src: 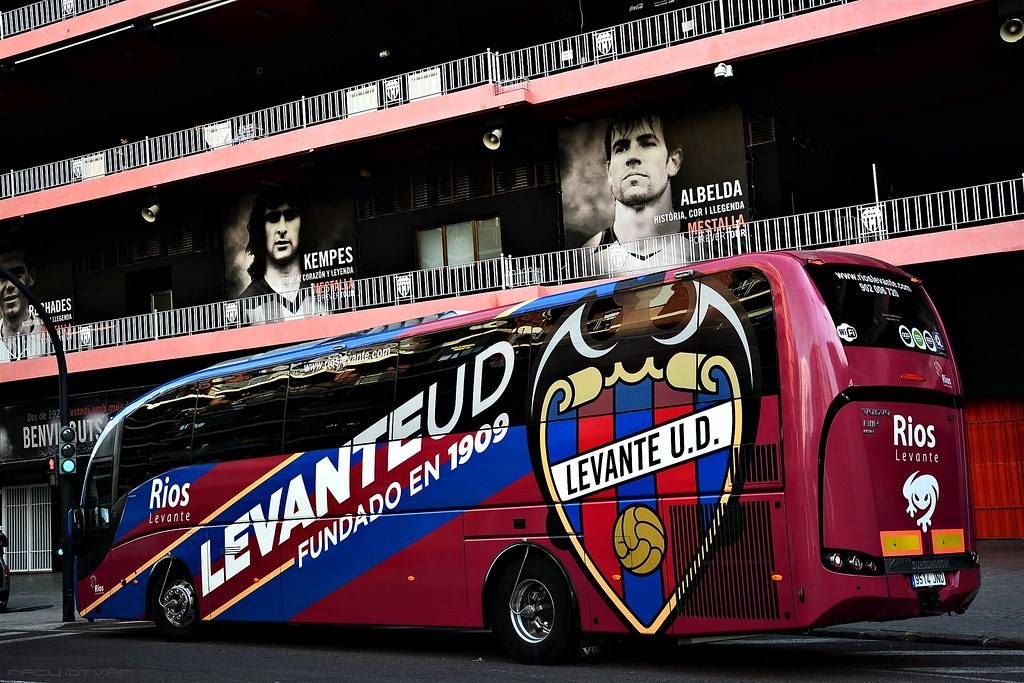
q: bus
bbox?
[67,250,982,663]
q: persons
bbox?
[575,104,716,279]
[238,184,332,326]
[0,250,46,363]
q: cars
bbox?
[0,530,10,609]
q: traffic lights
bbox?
[46,457,59,486]
[58,427,78,474]
[56,539,73,565]
